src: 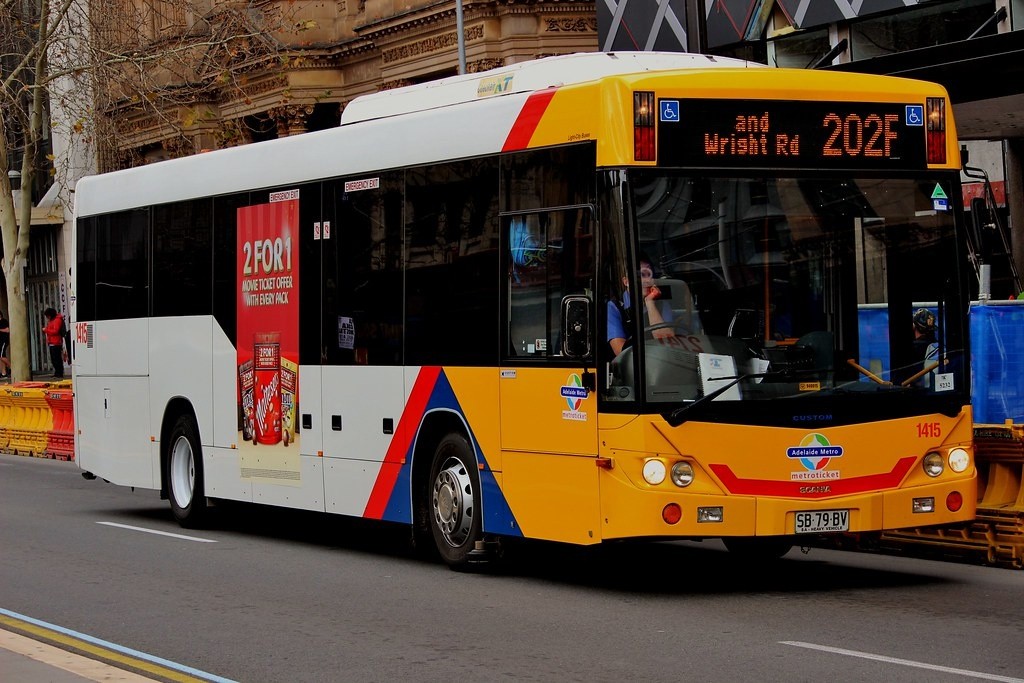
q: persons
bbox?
[913,309,948,389]
[605,260,674,356]
[0,311,12,378]
[41,308,67,381]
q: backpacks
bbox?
[58,318,66,337]
[921,341,947,389]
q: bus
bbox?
[70,51,1012,573]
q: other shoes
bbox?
[50,375,65,380]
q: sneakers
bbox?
[1,374,7,381]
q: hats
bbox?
[913,308,940,330]
